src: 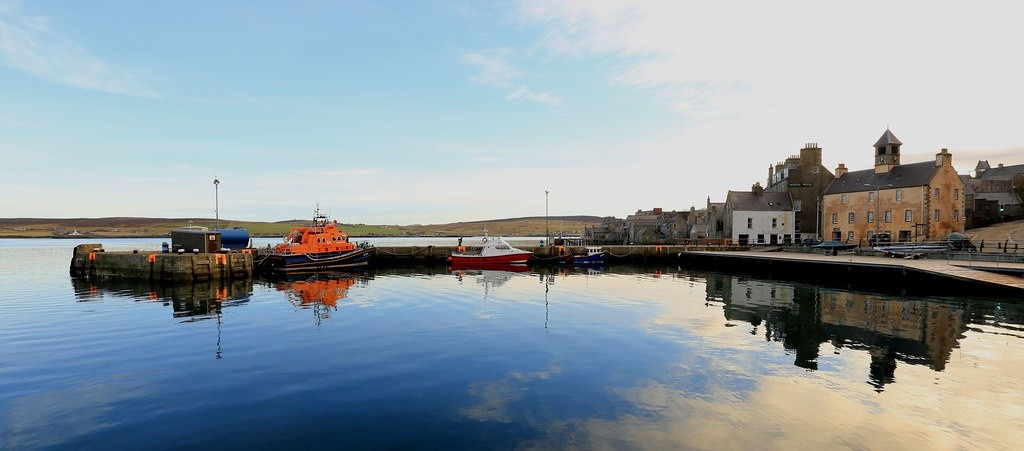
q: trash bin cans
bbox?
[162,242,169,253]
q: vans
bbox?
[870,233,891,247]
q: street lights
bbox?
[545,190,551,247]
[213,178,220,231]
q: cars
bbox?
[804,238,821,245]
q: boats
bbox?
[447,263,532,276]
[572,246,609,268]
[526,246,575,269]
[250,203,372,277]
[445,227,534,266]
